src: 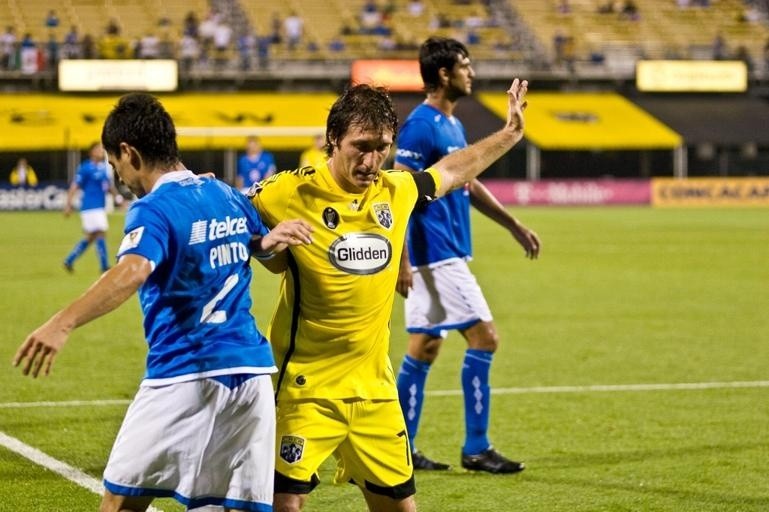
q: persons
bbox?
[64,142,129,275]
[1,1,769,216]
[14,95,289,512]
[392,37,542,473]
[245,78,528,510]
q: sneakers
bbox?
[412,448,449,469]
[461,444,525,473]
